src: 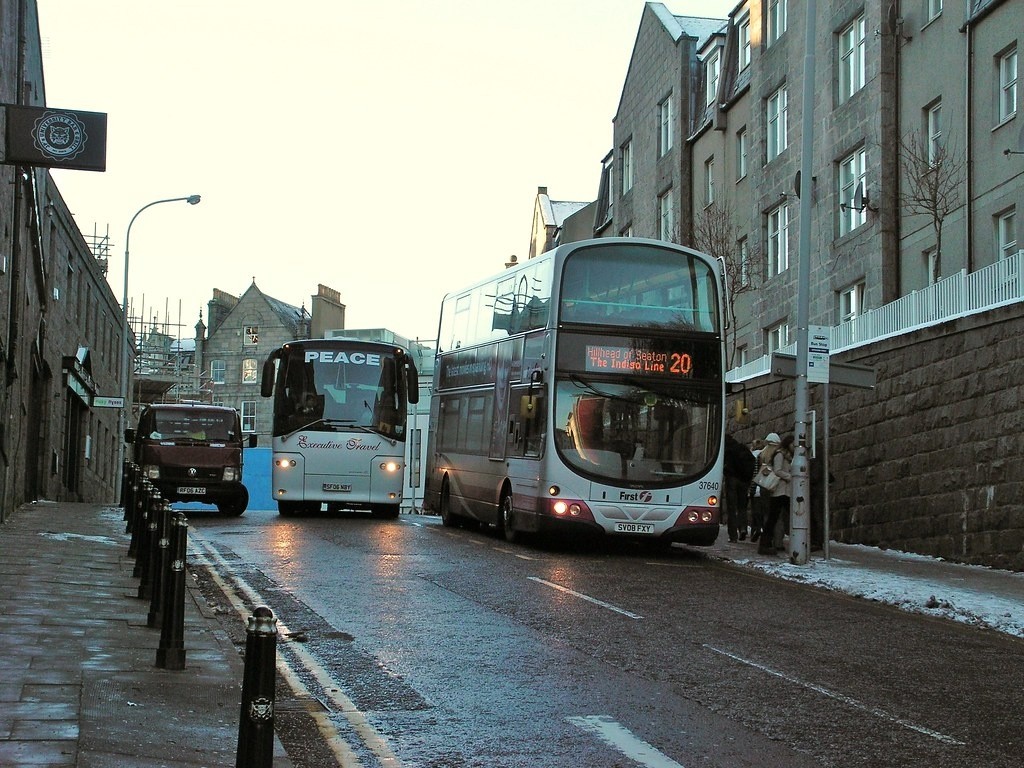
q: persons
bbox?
[724,432,756,542]
[750,433,795,555]
[298,395,318,416]
[810,441,834,553]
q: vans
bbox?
[125,403,257,517]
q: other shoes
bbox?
[809,541,823,552]
[775,547,786,551]
[728,537,737,543]
[739,529,747,541]
[758,545,777,555]
[751,529,761,542]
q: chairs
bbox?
[562,297,661,324]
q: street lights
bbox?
[113,195,201,504]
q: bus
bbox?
[260,337,418,520]
[423,236,749,560]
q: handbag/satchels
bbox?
[754,450,784,492]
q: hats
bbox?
[765,432,781,443]
